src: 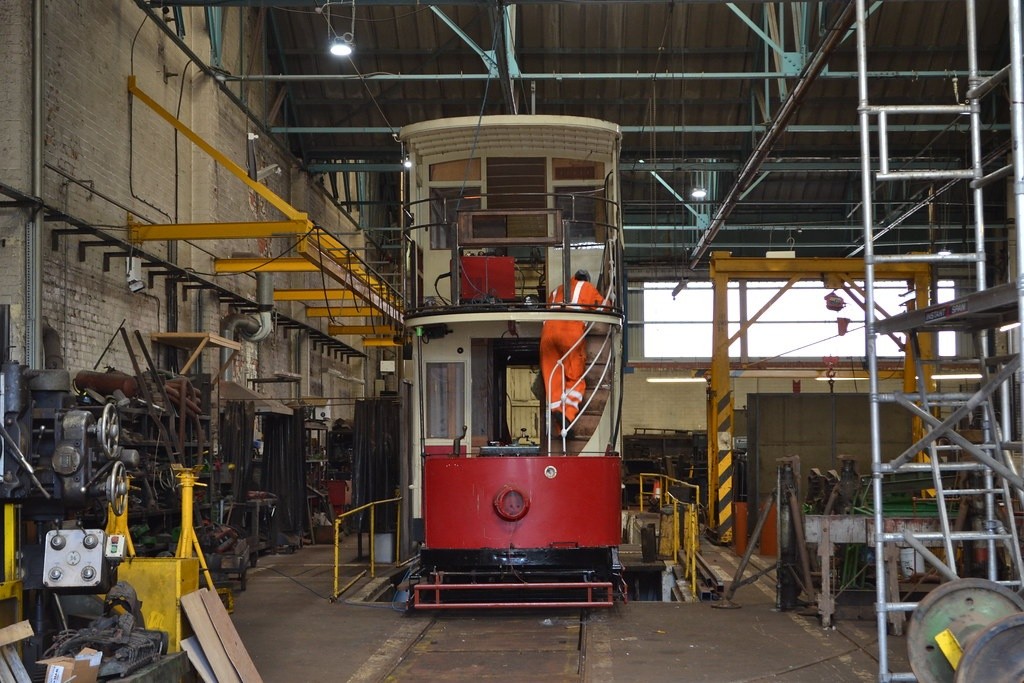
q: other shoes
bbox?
[554,410,575,441]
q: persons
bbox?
[653,477,660,510]
[540,269,612,440]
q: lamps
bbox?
[325,0,357,56]
[690,172,707,198]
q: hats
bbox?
[575,269,591,283]
